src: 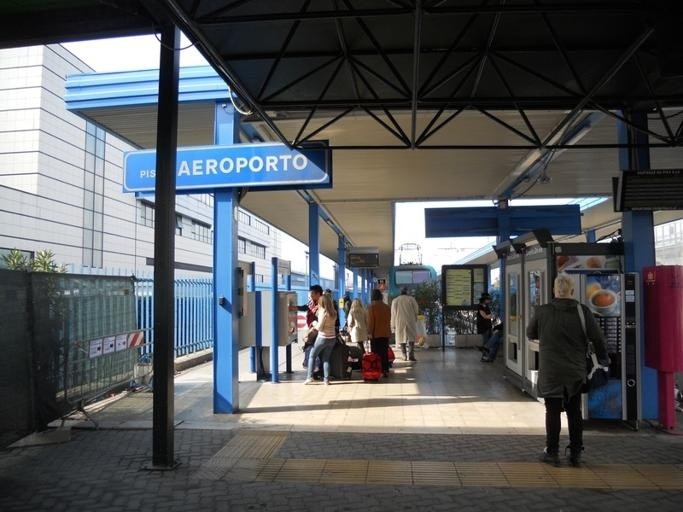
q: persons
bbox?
[289,285,419,386]
[526,273,610,466]
[477,292,494,359]
[476,320,504,362]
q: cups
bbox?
[582,256,606,269]
[586,283,601,298]
[589,288,621,315]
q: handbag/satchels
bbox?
[584,353,610,391]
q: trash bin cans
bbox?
[440,324,457,346]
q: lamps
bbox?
[495,121,592,210]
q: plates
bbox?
[561,256,582,268]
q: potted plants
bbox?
[448,292,499,349]
[414,278,442,348]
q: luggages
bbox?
[331,335,353,381]
[362,336,382,384]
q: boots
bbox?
[539,444,563,467]
[570,445,582,466]
